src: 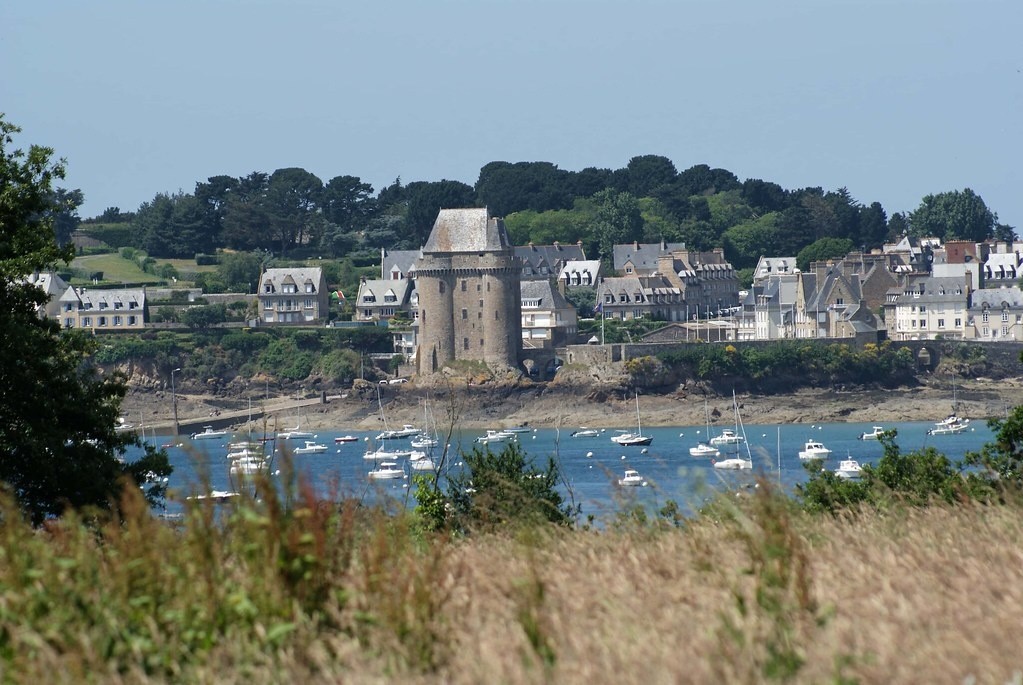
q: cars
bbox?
[379,379,388,385]
[701,306,741,319]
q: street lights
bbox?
[172,368,181,424]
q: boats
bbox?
[618,469,644,486]
[334,435,359,442]
[857,426,897,440]
[189,425,227,441]
[186,490,240,503]
[798,439,832,460]
[472,427,533,444]
[570,429,598,438]
[293,441,328,455]
[834,448,863,478]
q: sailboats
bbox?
[611,389,653,446]
[227,396,269,476]
[137,411,164,483]
[689,389,752,469]
[926,367,969,436]
[363,385,439,479]
[277,391,314,439]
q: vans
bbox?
[389,379,408,385]
